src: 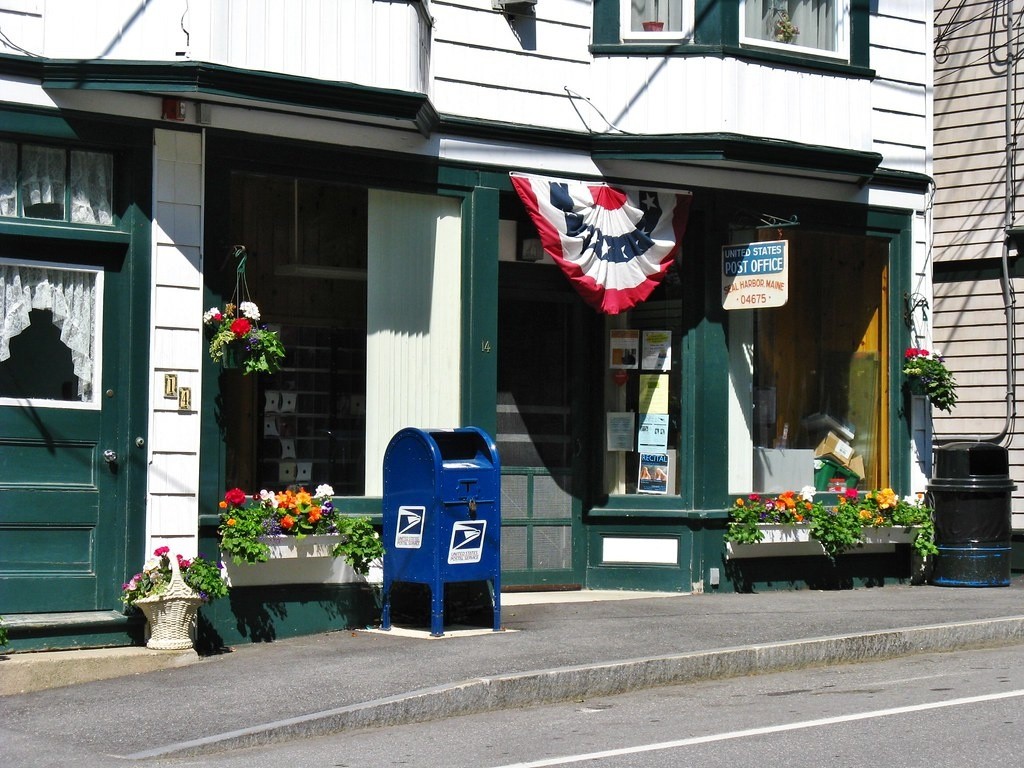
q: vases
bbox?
[131,595,204,649]
[219,339,253,368]
[909,372,931,396]
[728,516,924,544]
[227,531,348,561]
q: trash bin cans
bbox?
[925,441,1020,588]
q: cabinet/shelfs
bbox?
[753,274,820,448]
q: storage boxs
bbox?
[754,447,815,492]
[383,425,507,639]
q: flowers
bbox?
[218,484,352,532]
[902,348,961,415]
[202,300,289,374]
[775,13,801,43]
[728,489,926,526]
[119,546,229,608]
